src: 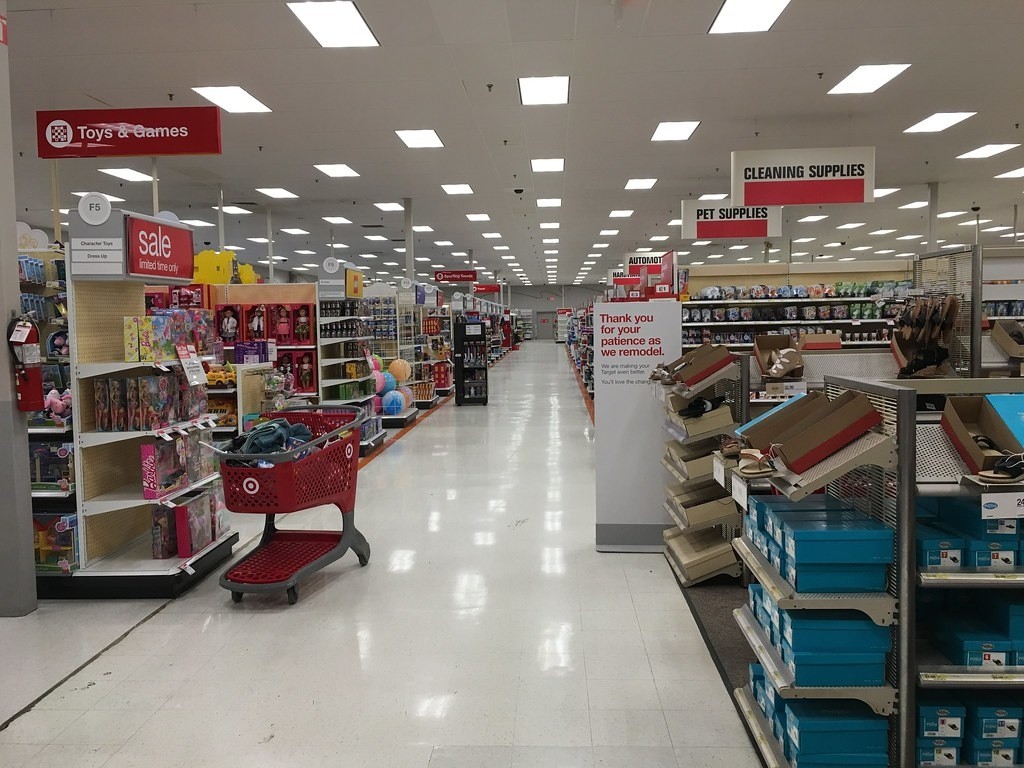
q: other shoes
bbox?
[678,395,725,417]
[649,362,670,380]
[662,361,692,384]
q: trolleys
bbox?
[217,404,371,605]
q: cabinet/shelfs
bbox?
[359,282,531,428]
[644,249,1024,353]
[731,375,1024,768]
[16,194,241,598]
[554,265,661,401]
[144,262,388,456]
[647,330,1023,590]
[203,362,321,439]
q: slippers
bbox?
[978,453,1024,484]
[718,438,745,457]
[894,296,959,344]
[972,434,1005,456]
[739,449,773,478]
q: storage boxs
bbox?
[798,334,841,351]
[734,391,1024,766]
[991,319,1024,356]
[7,254,230,574]
[649,341,737,579]
[890,331,951,378]
[752,335,805,381]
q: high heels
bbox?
[929,346,950,377]
[898,346,937,378]
[761,347,804,381]
